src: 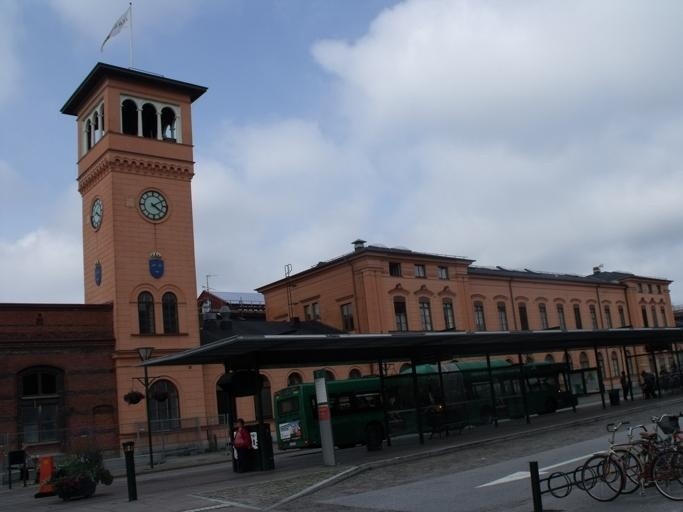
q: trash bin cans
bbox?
[607,389,621,406]
[228,421,276,474]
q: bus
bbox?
[273,357,580,453]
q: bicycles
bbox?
[582,411,681,503]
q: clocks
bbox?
[88,195,104,232]
[136,186,172,224]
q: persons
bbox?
[231,418,252,472]
[620,371,629,400]
[659,365,670,375]
[639,370,657,399]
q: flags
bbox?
[108,8,130,39]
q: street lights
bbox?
[137,345,156,467]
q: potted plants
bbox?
[123,390,145,404]
[151,390,170,402]
[45,458,114,503]
